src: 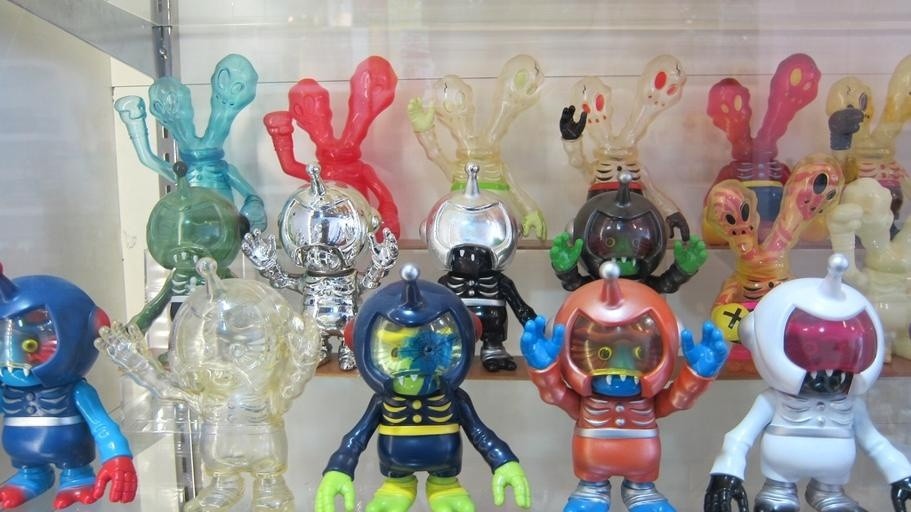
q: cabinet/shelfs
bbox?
[243,224,910,382]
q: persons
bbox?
[1,162,911,512]
